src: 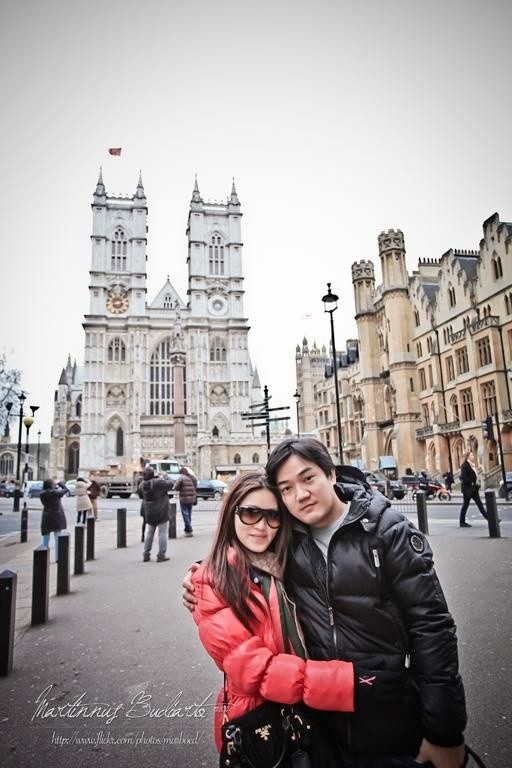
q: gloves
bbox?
[354,655,404,707]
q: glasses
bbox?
[234,505,282,529]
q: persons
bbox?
[190,469,354,767]
[459,452,501,527]
[39,464,197,563]
[442,468,455,493]
[182,437,468,768]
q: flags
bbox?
[108,148,122,156]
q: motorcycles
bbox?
[412,472,454,503]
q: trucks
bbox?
[90,458,183,500]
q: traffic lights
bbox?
[481,416,495,441]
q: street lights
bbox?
[21,415,36,542]
[322,281,347,466]
[3,391,41,513]
[293,388,302,439]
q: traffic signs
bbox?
[241,402,292,429]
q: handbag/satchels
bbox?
[220,698,310,767]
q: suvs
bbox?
[359,468,409,502]
[497,470,512,503]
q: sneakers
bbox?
[459,522,472,527]
[143,555,151,561]
[185,531,194,537]
[157,554,170,562]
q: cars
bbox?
[0,476,90,499]
[197,477,232,502]
